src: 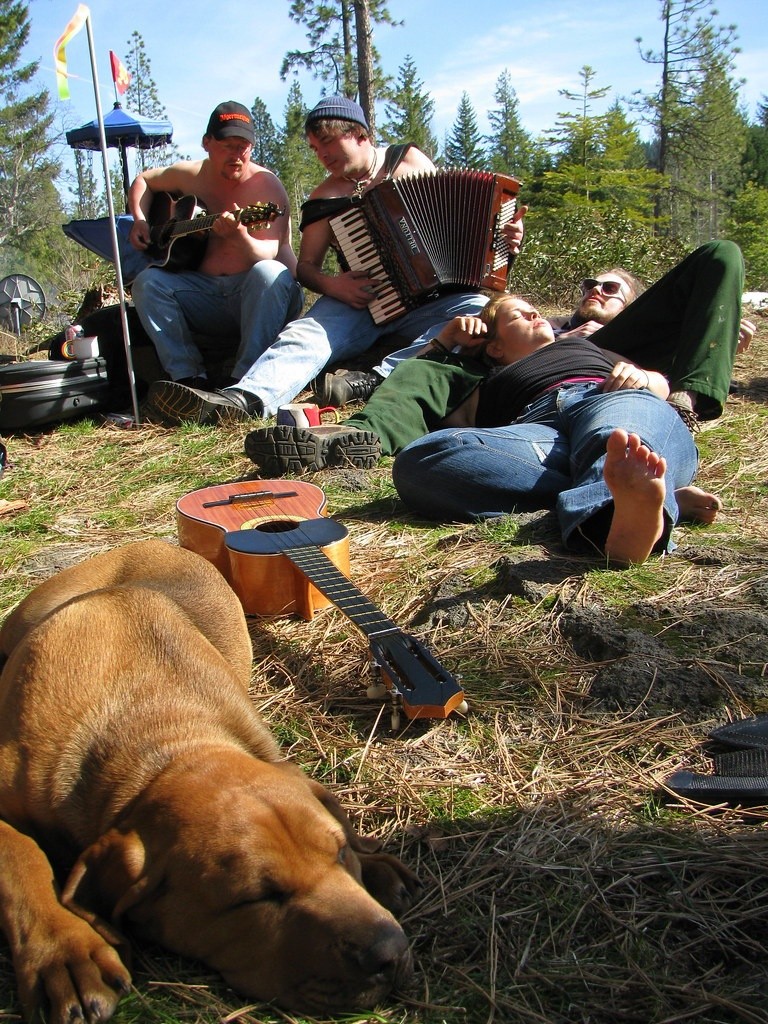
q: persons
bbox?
[127,99,757,568]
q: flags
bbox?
[109,50,130,94]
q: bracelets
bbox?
[641,367,650,390]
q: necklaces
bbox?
[342,146,377,196]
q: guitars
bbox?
[118,186,287,288]
[174,479,471,734]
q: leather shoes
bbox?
[324,369,380,408]
[147,381,250,426]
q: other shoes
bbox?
[244,424,382,476]
[667,401,701,441]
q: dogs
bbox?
[0,538,416,1024]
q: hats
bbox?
[305,96,369,132]
[208,101,255,146]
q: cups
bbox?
[62,335,100,360]
[276,403,341,428]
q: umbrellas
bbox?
[67,103,173,217]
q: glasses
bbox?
[583,278,628,307]
